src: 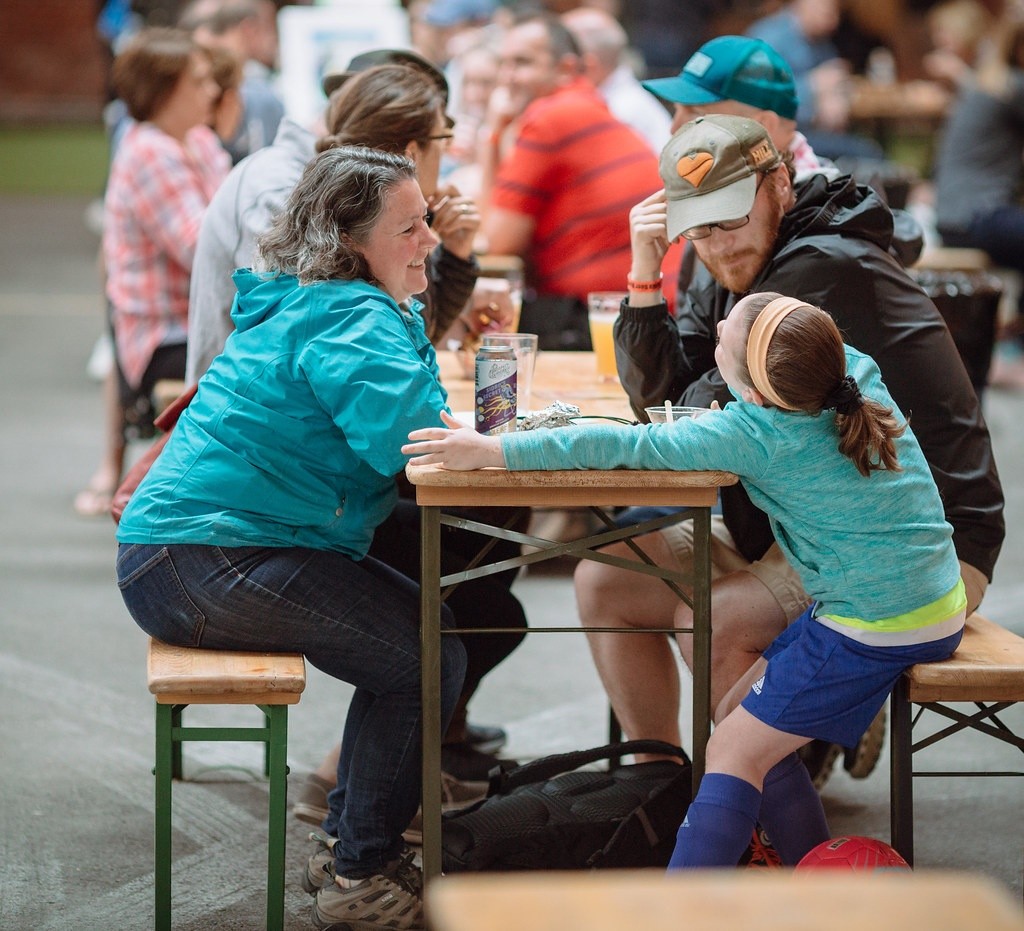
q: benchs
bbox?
[892,614,1023,865]
[148,633,307,931]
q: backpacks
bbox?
[440,739,694,872]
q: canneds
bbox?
[474,345,517,436]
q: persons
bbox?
[292,66,488,843]
[920,0,1024,272]
[185,49,527,785]
[639,33,887,792]
[573,113,1006,866]
[402,293,968,872]
[72,0,852,516]
[115,145,468,931]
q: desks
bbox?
[408,347,740,880]
[424,869,1024,931]
[847,80,943,152]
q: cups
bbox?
[643,406,712,424]
[585,291,637,381]
[482,334,538,419]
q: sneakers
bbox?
[292,774,424,845]
[310,876,424,931]
[746,824,786,871]
[417,769,490,815]
[301,831,423,898]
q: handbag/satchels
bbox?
[110,383,199,526]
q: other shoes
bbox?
[842,700,886,781]
[73,482,114,515]
[798,733,842,792]
[439,742,520,784]
[460,723,509,754]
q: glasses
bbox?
[679,171,765,240]
[398,126,454,154]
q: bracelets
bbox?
[627,272,664,293]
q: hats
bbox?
[322,49,455,129]
[641,35,799,120]
[659,114,779,243]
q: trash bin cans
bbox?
[909,269,1004,390]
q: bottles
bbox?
[468,256,522,363]
